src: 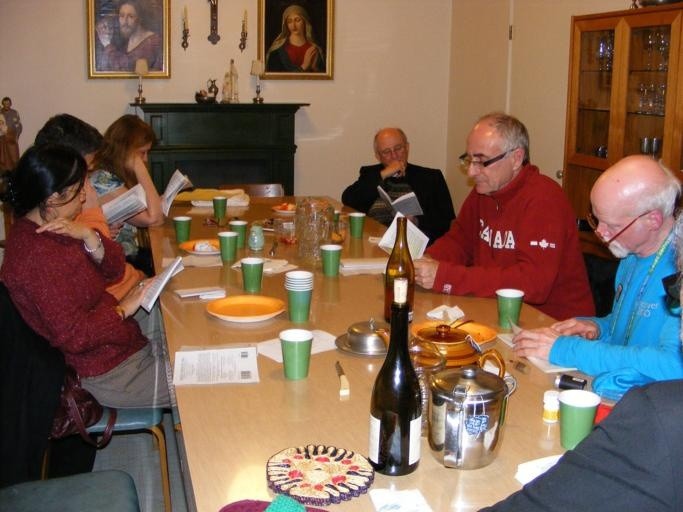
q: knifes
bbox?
[333,359,351,399]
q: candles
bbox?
[240,10,247,35]
[181,6,189,29]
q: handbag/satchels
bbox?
[48,376,102,439]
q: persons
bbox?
[97,3,162,72]
[267,5,326,72]
[467,206,683,512]
[513,154,681,389]
[1,97,22,145]
[412,113,597,324]
[3,144,173,409]
[35,111,164,336]
[342,126,455,245]
[89,114,165,275]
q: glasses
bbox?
[585,210,652,248]
[662,272,682,300]
[459,147,519,170]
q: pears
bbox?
[199,88,207,98]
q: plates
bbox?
[335,335,395,359]
[252,220,274,231]
[205,295,286,323]
[271,205,295,213]
[435,359,518,404]
[178,238,221,255]
[412,319,498,347]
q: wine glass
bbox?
[594,25,669,118]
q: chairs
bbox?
[0,467,143,511]
[580,248,623,317]
[0,279,174,511]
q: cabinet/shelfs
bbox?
[559,2,682,272]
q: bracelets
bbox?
[83,232,102,252]
[110,305,126,321]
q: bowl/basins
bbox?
[194,95,215,103]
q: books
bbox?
[140,254,184,314]
[497,326,580,375]
[376,183,423,217]
[376,211,430,262]
[176,287,226,299]
[160,168,192,217]
[174,341,258,387]
[232,256,298,275]
[518,453,567,487]
[101,181,147,226]
[256,328,337,363]
[368,486,430,511]
[339,256,389,275]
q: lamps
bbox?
[134,58,148,106]
[248,60,266,105]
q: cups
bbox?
[349,213,366,238]
[426,365,508,471]
[285,270,315,324]
[240,257,264,291]
[173,217,193,242]
[229,221,248,248]
[333,210,340,226]
[593,141,609,158]
[496,288,525,329]
[279,329,313,379]
[216,231,239,262]
[213,197,228,217]
[558,386,603,451]
[321,244,343,276]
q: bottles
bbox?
[364,275,426,478]
[296,197,331,263]
[639,136,652,156]
[541,390,558,423]
[648,136,661,156]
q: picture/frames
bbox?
[83,1,173,81]
[255,0,335,84]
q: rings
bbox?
[137,280,144,288]
